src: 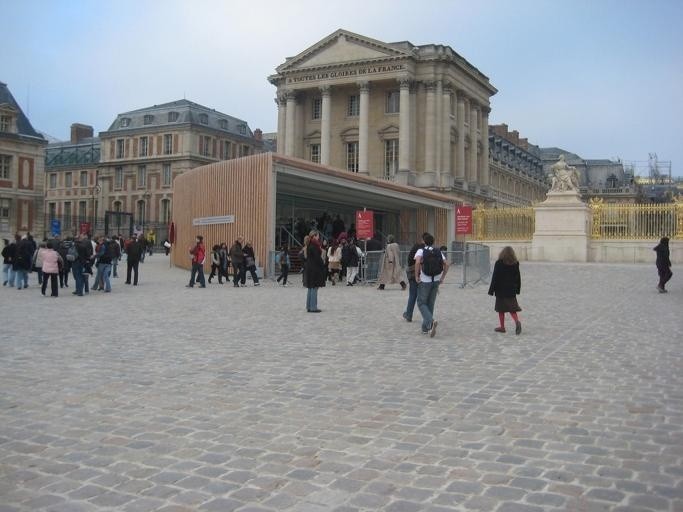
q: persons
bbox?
[184,235,205,288]
[207,244,223,284]
[232,240,260,287]
[228,235,248,288]
[278,212,383,286]
[652,237,672,293]
[487,246,521,335]
[413,234,449,338]
[0,225,156,299]
[403,232,429,322]
[303,228,324,313]
[217,243,230,281]
[276,244,290,287]
[375,234,406,290]
[550,154,580,194]
[162,238,171,256]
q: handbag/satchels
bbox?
[245,256,255,266]
[406,264,421,280]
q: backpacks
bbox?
[422,248,443,276]
[65,246,78,262]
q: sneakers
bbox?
[330,278,408,291]
[515,321,522,335]
[2,279,138,298]
[421,319,438,337]
[403,312,412,321]
[306,307,321,313]
[495,327,505,333]
[186,275,289,290]
